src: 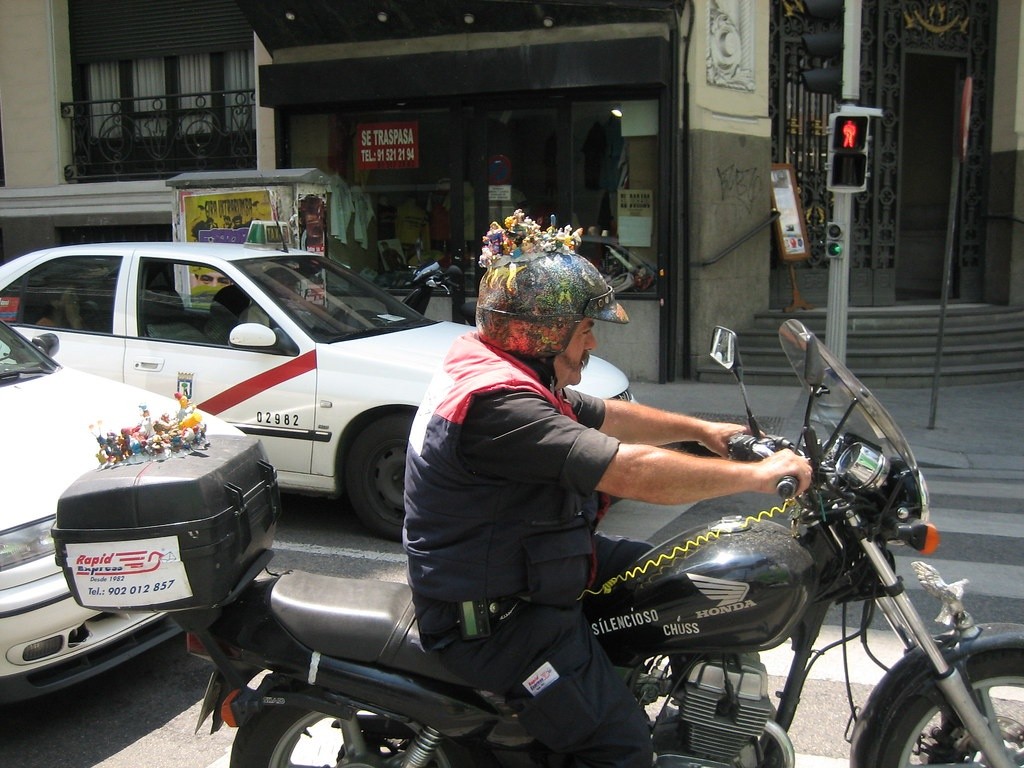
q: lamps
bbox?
[610,104,623,119]
[462,11,477,23]
[286,10,298,21]
[544,10,558,29]
[376,12,389,23]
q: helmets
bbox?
[475,208,630,391]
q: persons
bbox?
[34,271,89,331]
[380,241,403,268]
[403,249,812,768]
[377,177,529,258]
[240,266,330,355]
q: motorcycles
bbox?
[51,317,1023,768]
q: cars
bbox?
[1,241,640,543]
[463,237,657,294]
[0,317,247,707]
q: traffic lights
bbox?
[825,114,869,193]
[825,221,843,259]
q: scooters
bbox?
[401,238,478,327]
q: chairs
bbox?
[205,284,272,347]
[143,285,212,344]
